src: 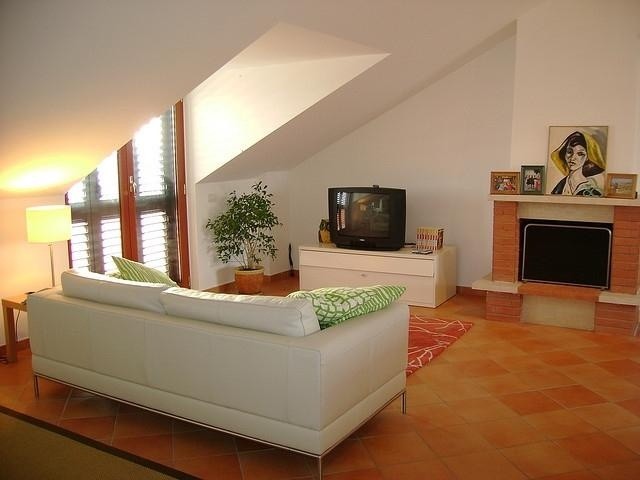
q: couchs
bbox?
[27,269,411,480]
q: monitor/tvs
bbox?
[328,187,406,251]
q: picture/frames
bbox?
[604,172,638,199]
[490,171,519,194]
[520,163,546,195]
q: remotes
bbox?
[411,250,432,255]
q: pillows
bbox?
[284,285,406,331]
[112,256,181,289]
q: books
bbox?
[415,226,444,250]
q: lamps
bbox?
[27,205,73,288]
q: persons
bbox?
[494,176,516,191]
[549,129,606,197]
[524,169,542,191]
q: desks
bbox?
[3,293,27,363]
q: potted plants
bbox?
[206,180,283,294]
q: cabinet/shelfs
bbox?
[299,241,456,309]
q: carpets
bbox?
[406,311,474,374]
[1,405,201,480]
